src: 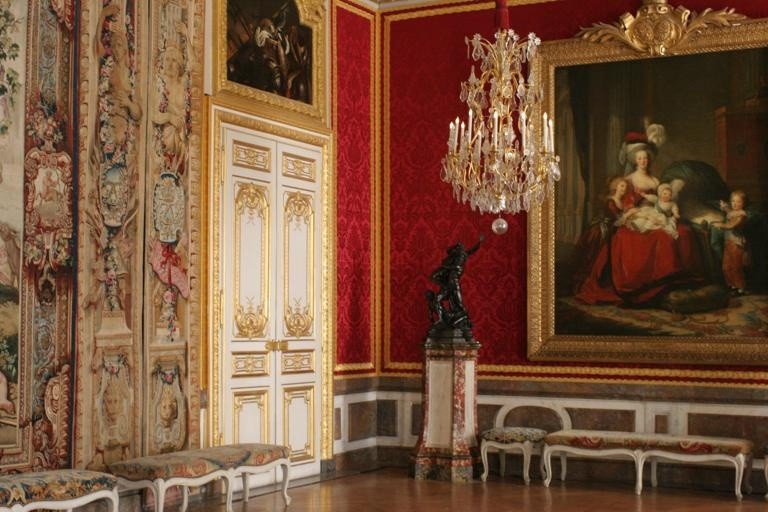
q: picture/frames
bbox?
[211,1,326,128]
[527,1,768,367]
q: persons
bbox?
[253,8,309,99]
[572,133,698,305]
[641,181,682,218]
[424,236,481,328]
[712,189,749,296]
[40,170,61,203]
[568,175,633,271]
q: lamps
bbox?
[439,1,561,234]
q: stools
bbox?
[480,426,547,484]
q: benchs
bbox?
[0,443,292,512]
[543,428,754,503]
